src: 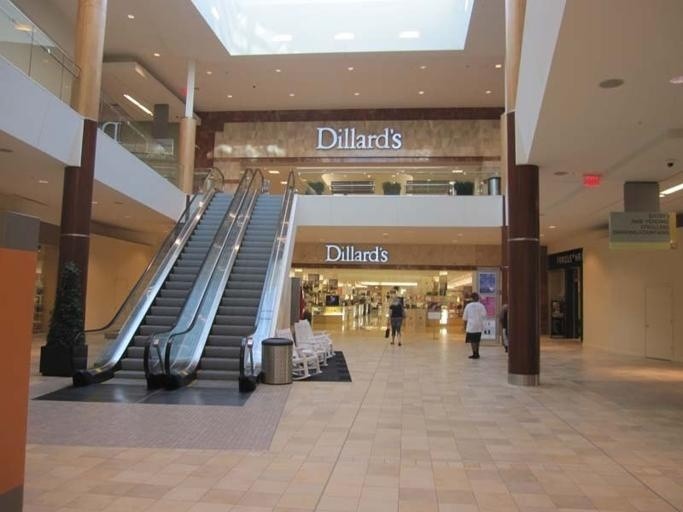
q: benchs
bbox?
[406,181,449,195]
[331,181,374,195]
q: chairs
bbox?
[276,318,335,379]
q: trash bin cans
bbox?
[488,177,500,195]
[262,338,293,385]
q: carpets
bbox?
[292,351,352,381]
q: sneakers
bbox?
[391,342,401,346]
[469,354,479,358]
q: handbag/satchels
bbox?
[386,329,389,337]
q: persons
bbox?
[461,290,487,359]
[498,304,508,352]
[387,294,404,348]
